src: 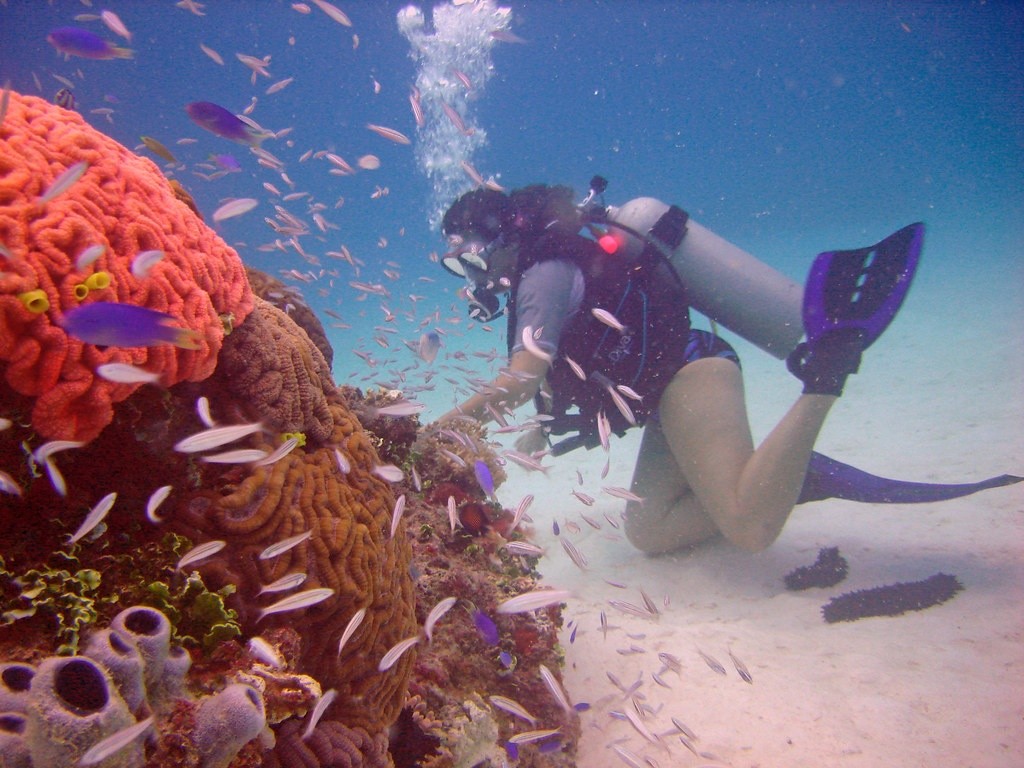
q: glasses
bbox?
[440,233,508,277]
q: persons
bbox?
[418,189,1024,552]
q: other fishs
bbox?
[1,2,756,768]
